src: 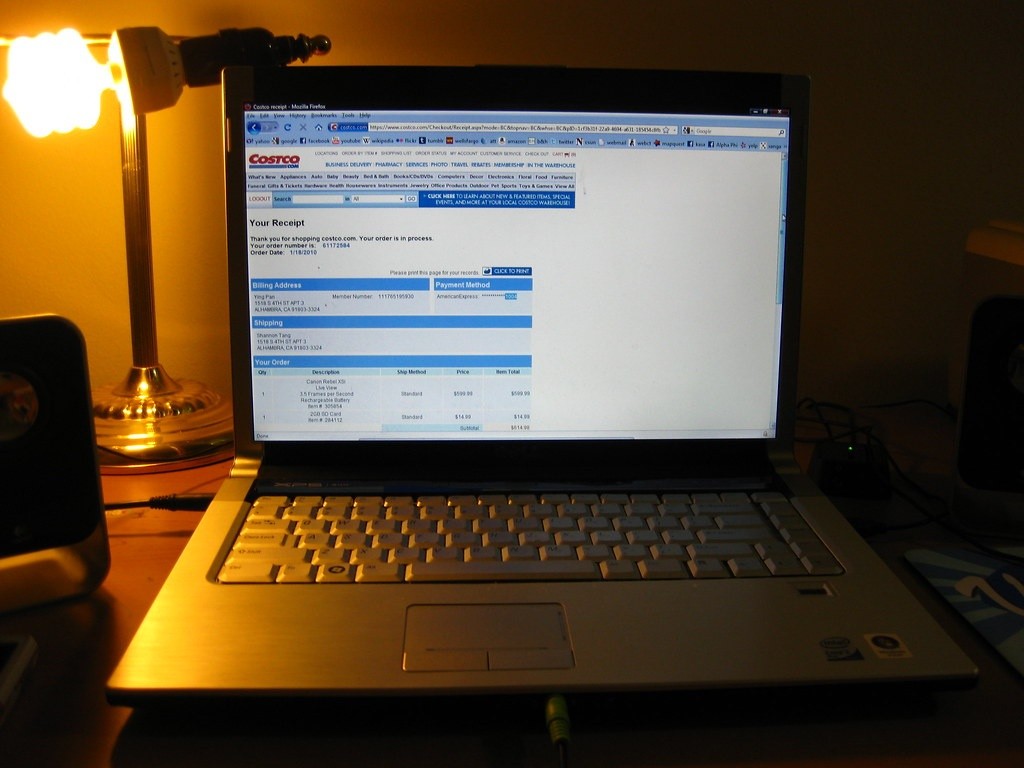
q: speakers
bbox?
[956,296,1024,540]
[0,316,113,612]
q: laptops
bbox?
[107,67,979,708]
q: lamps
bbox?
[0,25,333,462]
